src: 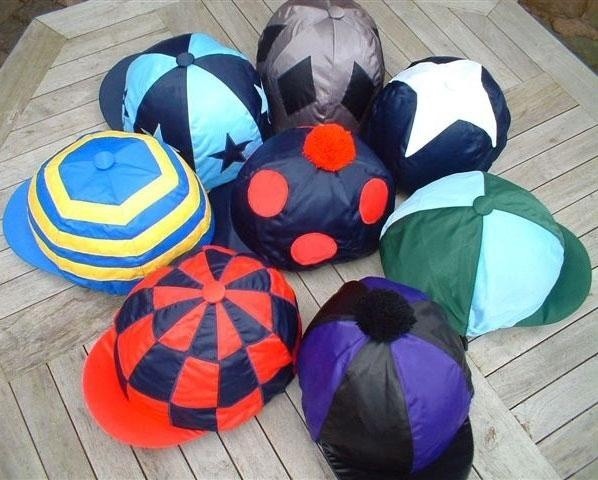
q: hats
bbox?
[256,0,384,135]
[2,129,216,295]
[379,170,592,337]
[208,123,396,273]
[98,32,273,191]
[296,276,475,480]
[82,244,303,447]
[358,56,511,196]
